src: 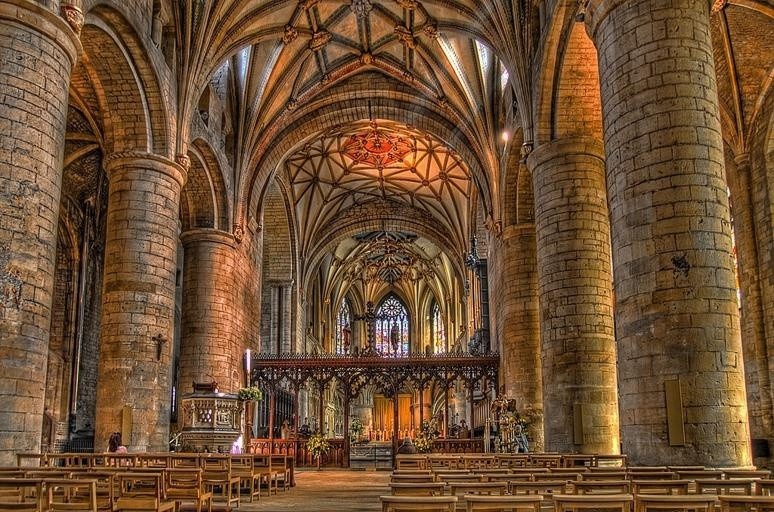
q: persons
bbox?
[107,433,127,466]
[514,424,529,452]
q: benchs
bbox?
[0,452,290,512]
[380,447,774,512]
